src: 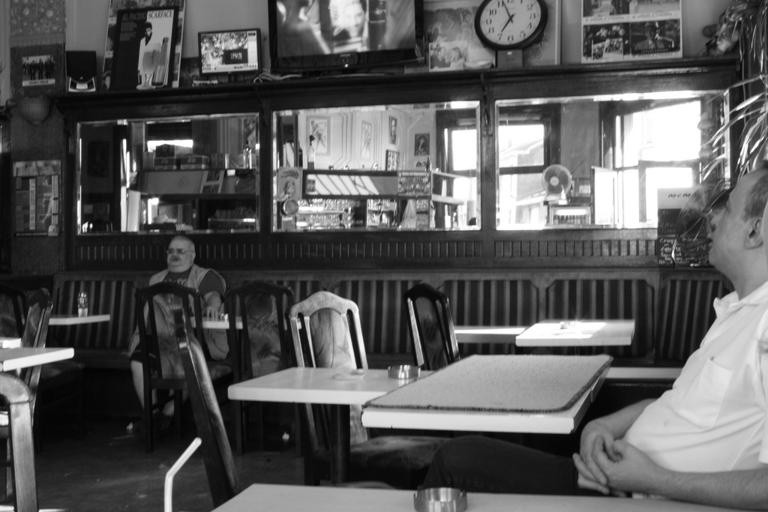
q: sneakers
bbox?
[125,410,175,442]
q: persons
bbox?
[635,22,674,55]
[430,46,466,71]
[125,233,226,435]
[422,159,767,511]
[341,0,366,37]
[23,58,55,80]
[138,22,162,88]
[584,24,627,58]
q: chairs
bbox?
[3,374,39,511]
[405,284,460,370]
[286,290,367,485]
[240,281,288,451]
[163,335,240,511]
[136,282,236,452]
[2,287,53,502]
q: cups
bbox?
[78,289,89,318]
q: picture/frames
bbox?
[196,27,263,75]
[384,149,400,172]
[7,43,66,97]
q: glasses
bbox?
[166,248,194,254]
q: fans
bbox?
[541,164,574,207]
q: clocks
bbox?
[474,1,548,50]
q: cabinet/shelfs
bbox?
[9,160,61,237]
[138,167,306,234]
[394,169,463,231]
[295,194,399,231]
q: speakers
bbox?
[66,50,98,89]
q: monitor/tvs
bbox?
[198,28,261,86]
[268,0,423,71]
